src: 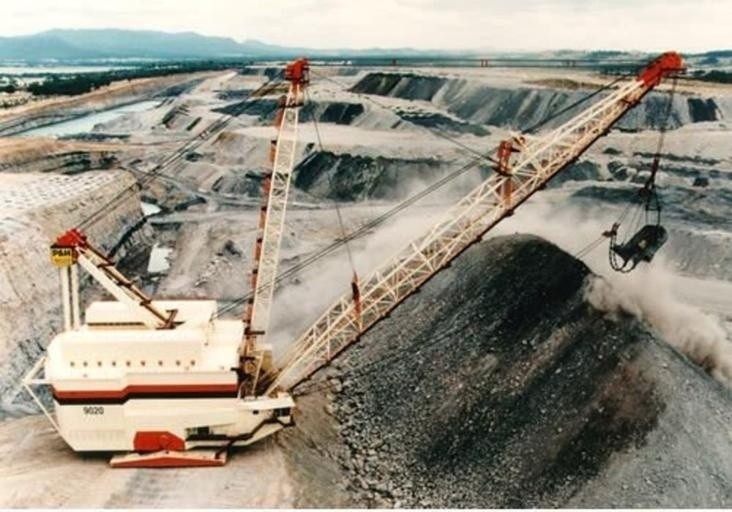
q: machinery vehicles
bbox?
[21,52,687,468]
